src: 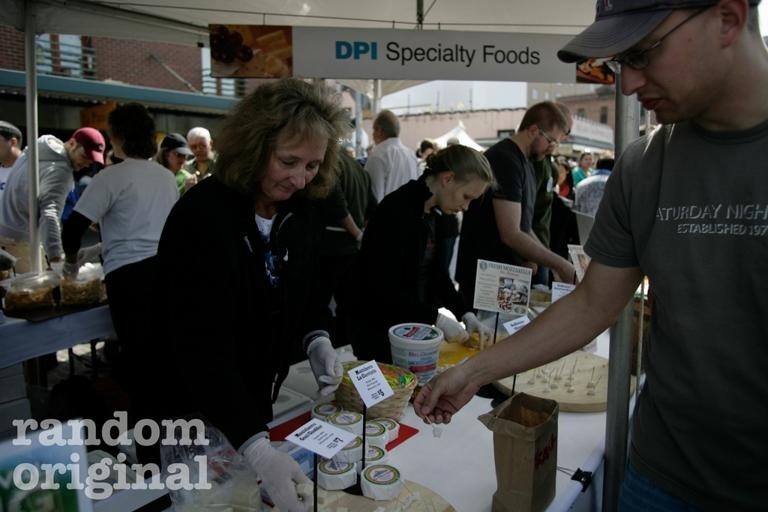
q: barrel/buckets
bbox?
[389,323,443,386]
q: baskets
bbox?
[337,361,417,419]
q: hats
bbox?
[558,0,760,63]
[0,121,23,137]
[161,133,194,156]
[74,127,105,166]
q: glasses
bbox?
[538,128,560,149]
[604,10,699,75]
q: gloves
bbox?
[246,437,314,511]
[63,261,79,281]
[462,313,494,352]
[437,307,469,343]
[77,244,99,267]
[307,336,345,397]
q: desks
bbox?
[0,303,118,439]
[94,288,648,512]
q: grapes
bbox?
[210,25,254,64]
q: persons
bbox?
[0,1,767,512]
